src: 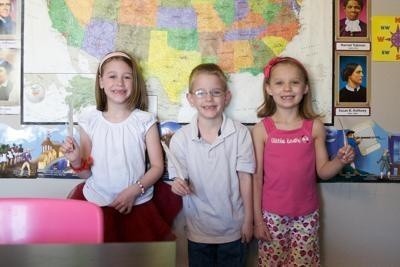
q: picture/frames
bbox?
[0,0,21,40]
[334,50,371,108]
[334,0,371,43]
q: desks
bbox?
[0,241,177,267]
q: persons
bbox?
[0,0,16,35]
[0,57,13,100]
[168,64,257,267]
[0,144,32,177]
[59,51,165,242]
[251,57,355,267]
[339,56,366,102]
[376,149,392,179]
[339,0,368,38]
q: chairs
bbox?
[0,197,104,244]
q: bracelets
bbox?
[70,158,87,172]
[253,221,265,230]
[134,180,144,197]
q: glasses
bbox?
[190,88,226,97]
